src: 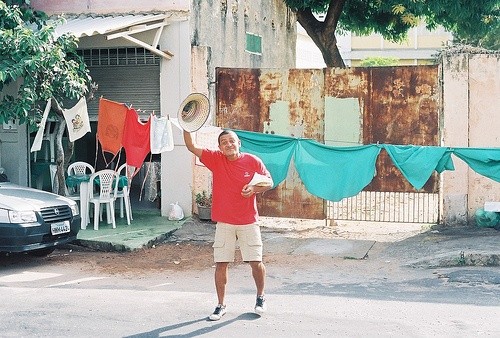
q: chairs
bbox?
[65,161,136,230]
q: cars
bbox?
[0,173,80,257]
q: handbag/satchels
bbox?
[169,201,184,220]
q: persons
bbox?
[183,128,273,321]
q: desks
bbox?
[65,175,131,229]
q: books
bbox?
[247,172,273,196]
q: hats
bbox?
[177,92,210,133]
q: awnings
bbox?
[21,11,169,38]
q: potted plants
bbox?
[194,191,212,219]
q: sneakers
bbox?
[209,304,227,321]
[254,298,265,316]
